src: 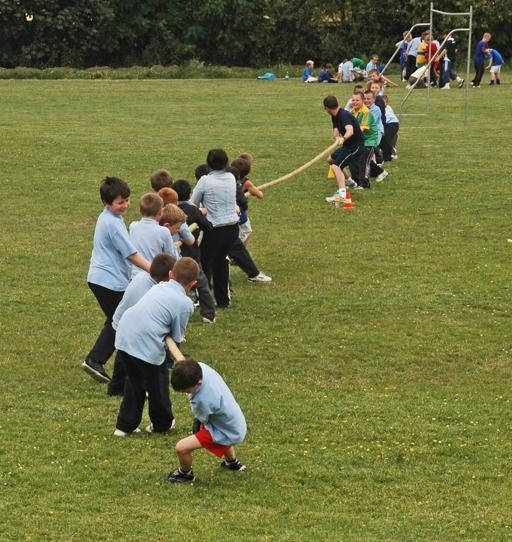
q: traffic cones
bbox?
[327,164,335,179]
[343,191,354,208]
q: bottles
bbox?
[335,191,340,208]
[285,70,289,80]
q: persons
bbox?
[470,32,491,88]
[303,29,465,202]
[81,149,272,439]
[164,361,248,482]
[484,48,504,85]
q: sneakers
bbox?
[325,194,346,203]
[219,460,245,472]
[146,419,176,433]
[80,358,112,383]
[377,146,398,167]
[246,273,274,283]
[163,468,196,484]
[114,428,142,437]
[441,78,481,89]
[346,177,371,191]
[375,169,388,182]
[193,279,233,324]
